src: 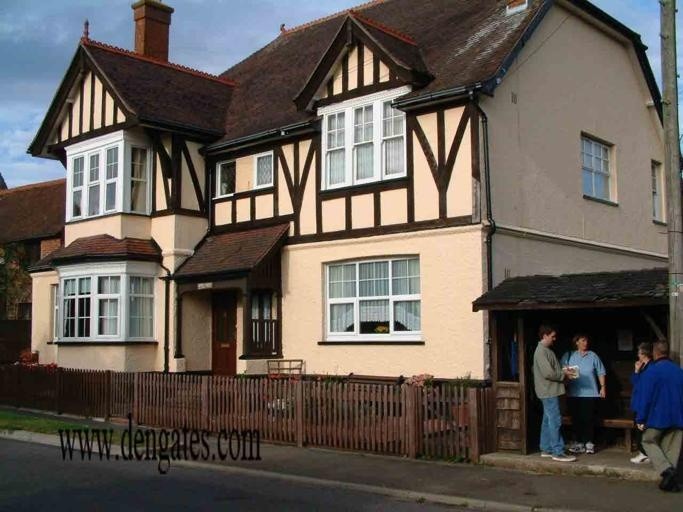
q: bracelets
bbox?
[601,384,606,387]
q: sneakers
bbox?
[541,442,594,462]
[630,452,649,463]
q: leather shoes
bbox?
[660,469,683,490]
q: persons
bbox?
[629,340,683,493]
[629,342,653,464]
[559,332,607,454]
[533,325,577,462]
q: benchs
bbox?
[560,414,635,455]
